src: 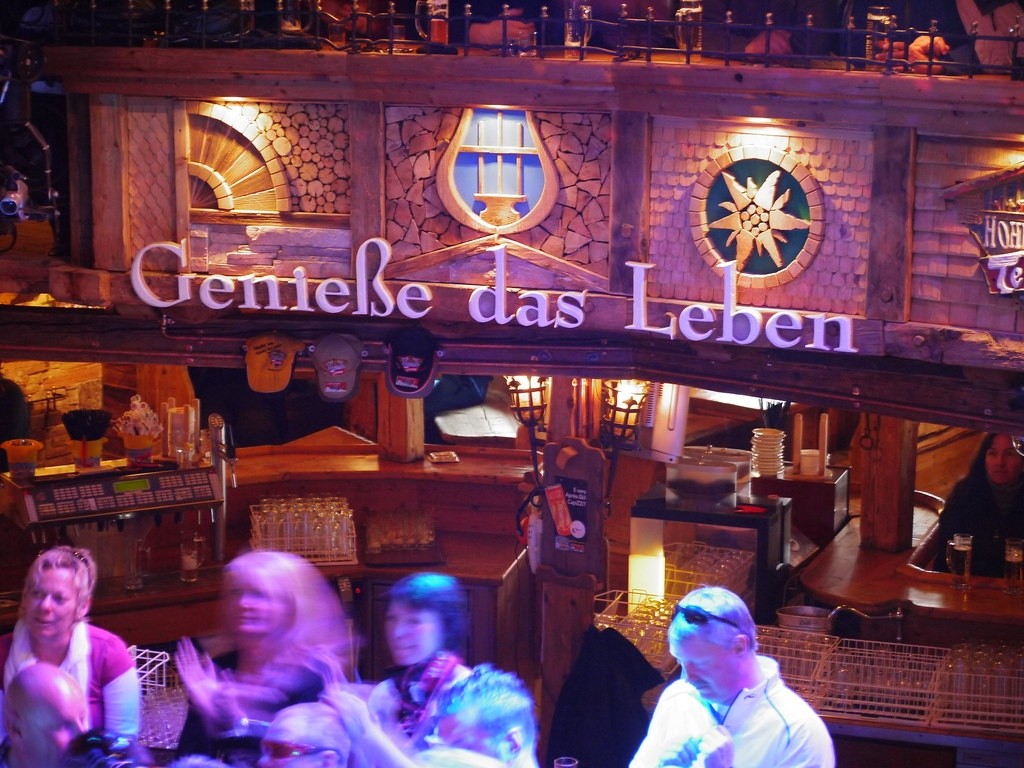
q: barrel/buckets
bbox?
[776,606,832,645]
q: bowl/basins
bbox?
[801,449,819,475]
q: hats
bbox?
[241,329,305,403]
[382,327,437,399]
[311,331,364,403]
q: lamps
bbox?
[499,372,656,521]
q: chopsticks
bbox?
[60,408,112,441]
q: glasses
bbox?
[671,603,741,629]
[72,728,145,766]
[260,739,344,765]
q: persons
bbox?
[3,546,140,743]
[447,0,566,46]
[848,0,981,76]
[937,432,1024,578]
[169,553,541,768]
[0,662,87,768]
[630,586,835,768]
[701,0,838,62]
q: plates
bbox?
[751,428,786,477]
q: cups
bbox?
[120,534,152,591]
[328,23,345,48]
[519,31,537,56]
[946,534,973,591]
[170,530,208,589]
[941,642,1024,710]
[70,437,108,471]
[390,25,404,50]
[554,756,578,767]
[122,434,154,465]
[415,1,450,45]
[564,7,592,58]
[364,508,436,554]
[1,438,44,486]
[1001,537,1024,596]
[675,0,701,62]
[176,446,192,464]
[866,6,891,71]
[259,496,353,562]
